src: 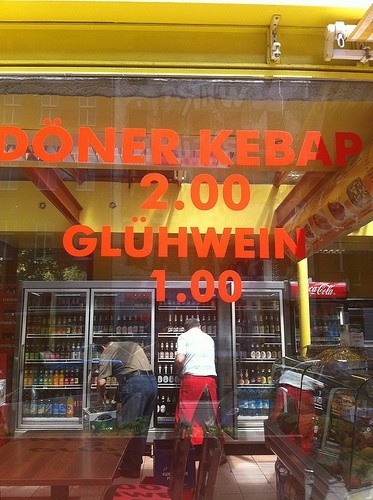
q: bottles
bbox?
[22,294,86,419]
[90,290,151,414]
[157,291,217,427]
[296,305,339,339]
[235,300,282,415]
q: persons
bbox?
[273,371,323,454]
[175,319,227,465]
[97,337,158,455]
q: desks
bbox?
[0,430,135,500]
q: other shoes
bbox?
[115,466,141,479]
[206,455,228,472]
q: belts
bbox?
[118,370,153,383]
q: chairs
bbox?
[104,427,224,500]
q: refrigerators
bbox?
[287,281,348,355]
[11,279,292,445]
